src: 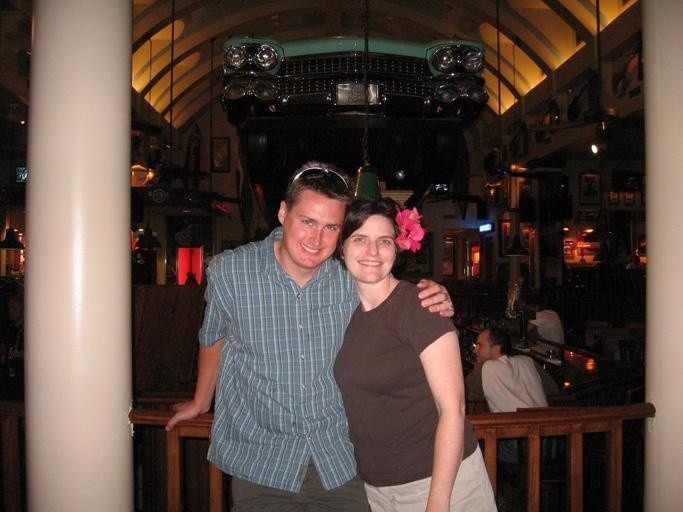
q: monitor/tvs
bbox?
[15,165,28,182]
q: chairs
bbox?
[499,323,645,511]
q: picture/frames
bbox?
[579,171,602,205]
[210,137,231,173]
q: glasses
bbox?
[292,167,349,189]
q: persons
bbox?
[465,329,563,495]
[331,197,498,512]
[165,167,456,512]
[524,291,568,358]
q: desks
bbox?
[463,327,641,407]
